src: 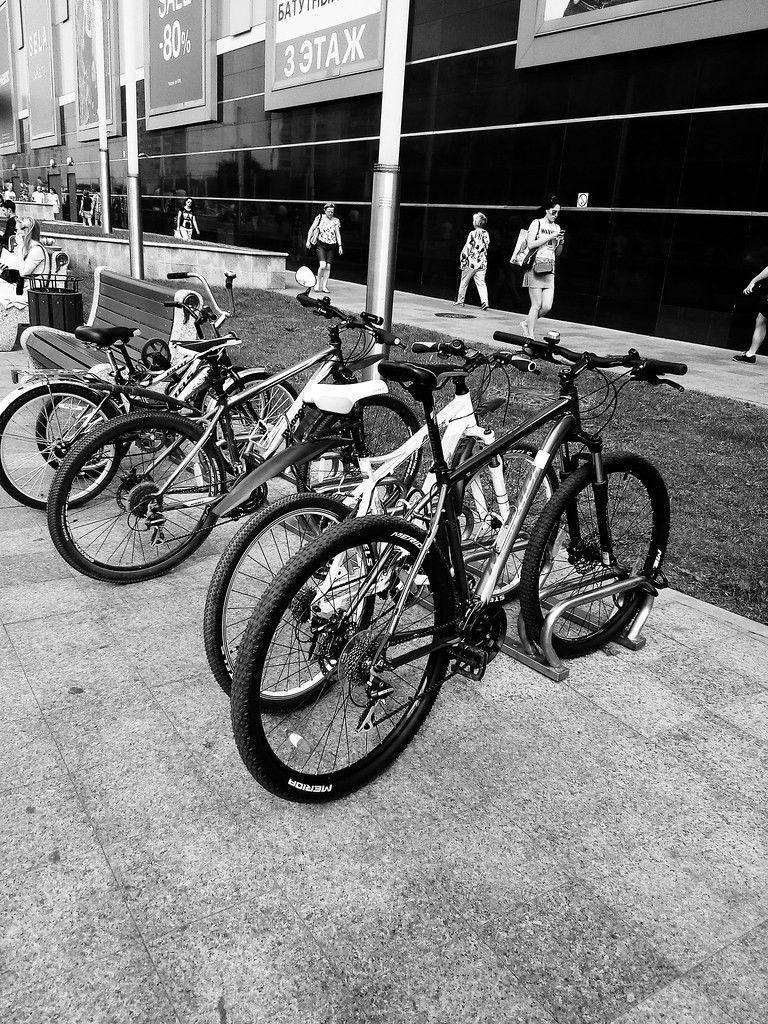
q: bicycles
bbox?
[0,268,691,805]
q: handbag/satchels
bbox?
[509,218,541,268]
[533,262,553,275]
[310,215,322,245]
[0,269,24,296]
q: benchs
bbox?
[20,266,205,446]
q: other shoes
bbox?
[453,303,464,307]
[519,322,528,336]
[322,288,330,293]
[314,285,319,292]
[482,302,489,309]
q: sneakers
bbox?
[733,355,757,364]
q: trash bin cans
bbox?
[23,273,85,333]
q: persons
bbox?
[79,190,94,226]
[519,195,566,341]
[732,266,768,365]
[3,182,16,202]
[45,187,61,213]
[90,187,102,227]
[176,198,200,239]
[0,215,52,311]
[453,212,490,310]
[31,185,45,204]
[0,200,18,253]
[306,202,343,293]
